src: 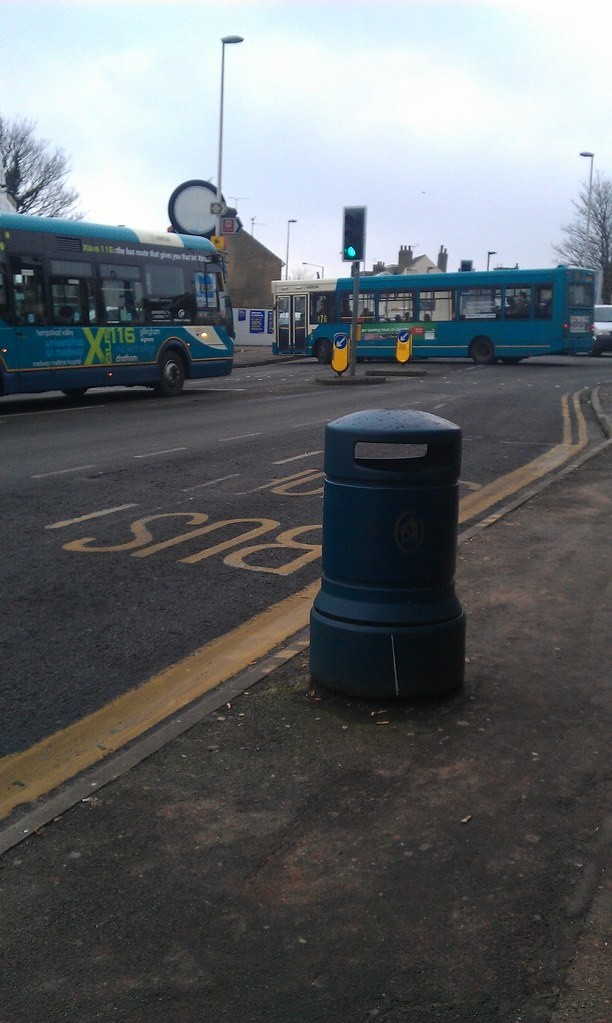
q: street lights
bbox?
[285,219,297,280]
[302,263,324,278]
[215,35,245,249]
[487,251,497,270]
[580,151,594,267]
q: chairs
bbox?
[397,315,401,322]
[405,312,411,321]
[424,314,431,321]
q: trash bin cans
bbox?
[305,406,469,705]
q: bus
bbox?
[271,265,597,366]
[1,211,235,398]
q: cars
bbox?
[75,305,144,324]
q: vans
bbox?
[594,305,612,357]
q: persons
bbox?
[317,299,327,315]
[406,312,409,319]
[59,306,74,323]
[343,304,353,316]
[505,293,529,317]
[360,308,368,319]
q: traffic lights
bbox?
[342,206,367,262]
